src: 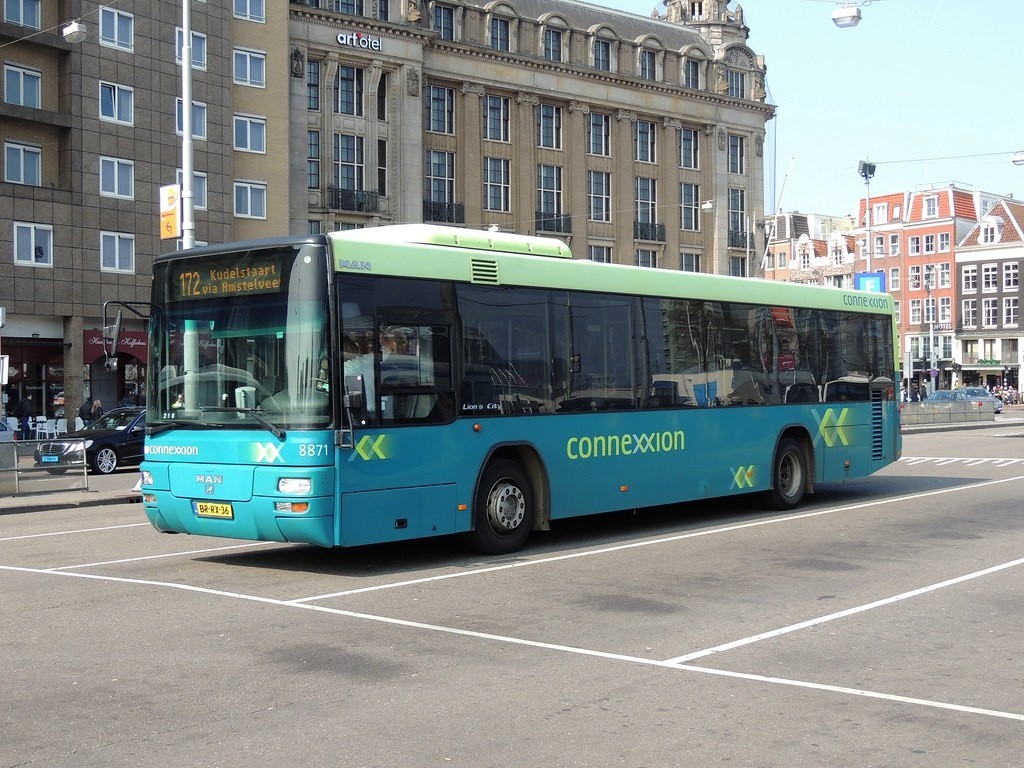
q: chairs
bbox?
[36,416,48,440]
[38,419,56,438]
[75,416,85,431]
[382,374,421,419]
[55,418,68,435]
[7,417,25,440]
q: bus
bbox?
[102,224,902,553]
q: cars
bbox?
[923,390,975,402]
[959,387,1002,414]
[34,406,147,475]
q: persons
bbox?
[901,379,1024,405]
[16,393,36,447]
[343,326,408,417]
[90,399,105,430]
[79,397,93,427]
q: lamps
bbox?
[702,199,713,212]
[831,1,872,28]
[487,223,499,232]
[62,20,87,44]
